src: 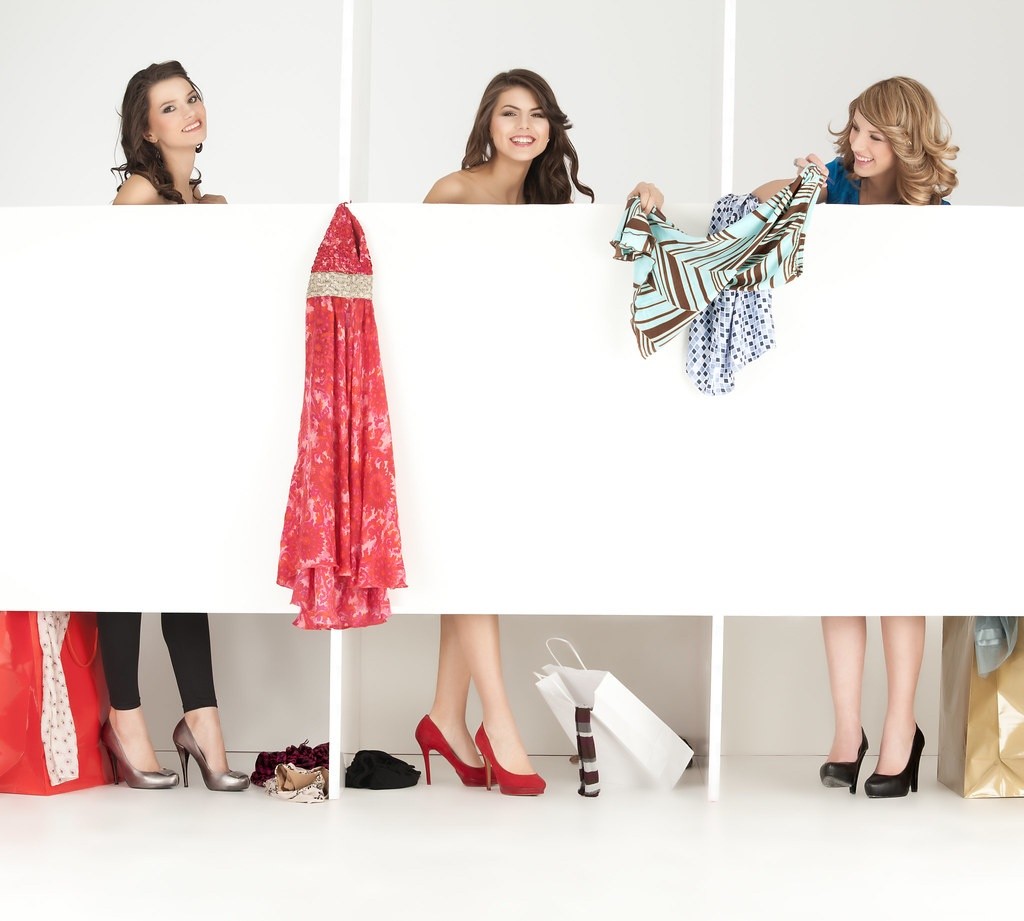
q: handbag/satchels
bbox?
[936,616,1024,799]
[531,637,695,792]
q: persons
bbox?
[93,61,250,792]
[413,68,662,799]
[753,76,960,799]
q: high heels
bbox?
[864,722,926,798]
[173,715,250,791]
[415,714,498,788]
[475,721,546,796]
[819,727,869,794]
[100,715,180,789]
